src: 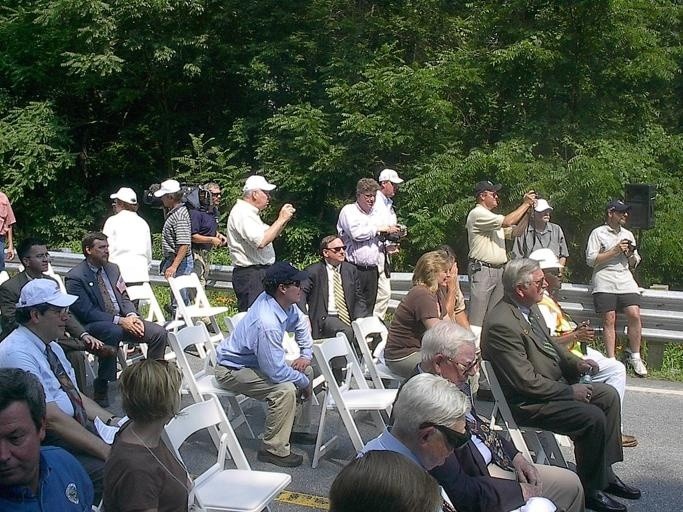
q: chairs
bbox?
[118,281,177,363]
[351,316,403,422]
[82,349,96,384]
[162,394,293,512]
[168,272,230,358]
[311,332,399,470]
[541,430,570,469]
[226,307,326,406]
[167,321,255,460]
[476,361,549,467]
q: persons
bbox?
[298,236,390,387]
[215,262,318,468]
[586,200,646,376]
[0,191,189,512]
[227,175,296,312]
[103,180,227,356]
[512,199,569,302]
[466,181,537,327]
[327,245,642,511]
[337,169,407,382]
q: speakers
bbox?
[624,183,656,231]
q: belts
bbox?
[344,260,378,270]
[469,259,506,269]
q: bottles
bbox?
[579,367,593,402]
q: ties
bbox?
[333,267,351,326]
[465,414,512,470]
[97,268,115,314]
[46,345,87,429]
[529,315,561,365]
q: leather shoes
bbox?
[604,476,641,499]
[584,490,627,511]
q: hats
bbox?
[605,200,631,211]
[243,175,277,191]
[527,248,564,268]
[474,180,502,197]
[110,187,137,204]
[379,169,404,183]
[154,179,180,198]
[16,277,79,308]
[533,199,553,213]
[262,263,307,285]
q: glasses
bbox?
[531,278,545,283]
[486,193,497,198]
[362,194,376,198]
[541,268,559,274]
[140,359,168,372]
[445,357,479,376]
[49,306,70,314]
[419,422,472,448]
[26,254,49,258]
[211,193,222,196]
[325,246,346,250]
[285,281,300,287]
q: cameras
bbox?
[523,189,540,208]
[290,203,299,217]
[621,239,638,256]
[379,225,408,246]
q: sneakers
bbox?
[290,433,318,445]
[258,450,302,466]
[208,323,220,332]
[94,378,109,408]
[184,344,206,357]
[621,436,636,446]
[629,357,647,376]
[163,304,177,317]
